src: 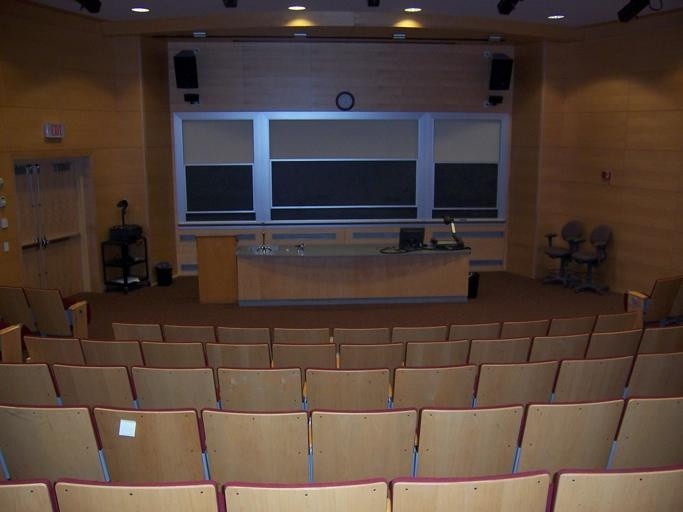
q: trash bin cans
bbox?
[468,271,479,299]
[155,262,173,286]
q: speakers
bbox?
[173,50,198,88]
[489,52,513,90]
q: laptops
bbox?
[400,227,425,250]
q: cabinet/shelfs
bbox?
[100,237,150,293]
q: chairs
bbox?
[572,225,612,296]
[0,285,90,339]
[542,221,584,288]
[622,276,682,322]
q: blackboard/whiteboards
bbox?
[433,162,500,221]
[270,160,420,221]
[184,166,257,224]
